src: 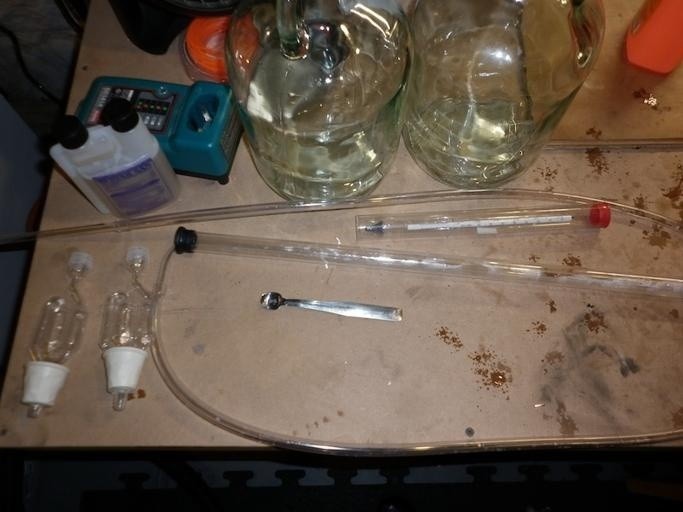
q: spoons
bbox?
[256,291,405,324]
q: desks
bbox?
[0,1,681,467]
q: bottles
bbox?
[624,0,683,76]
[224,0,417,206]
[46,95,181,222]
[400,0,609,191]
[352,200,613,244]
[173,224,683,304]
